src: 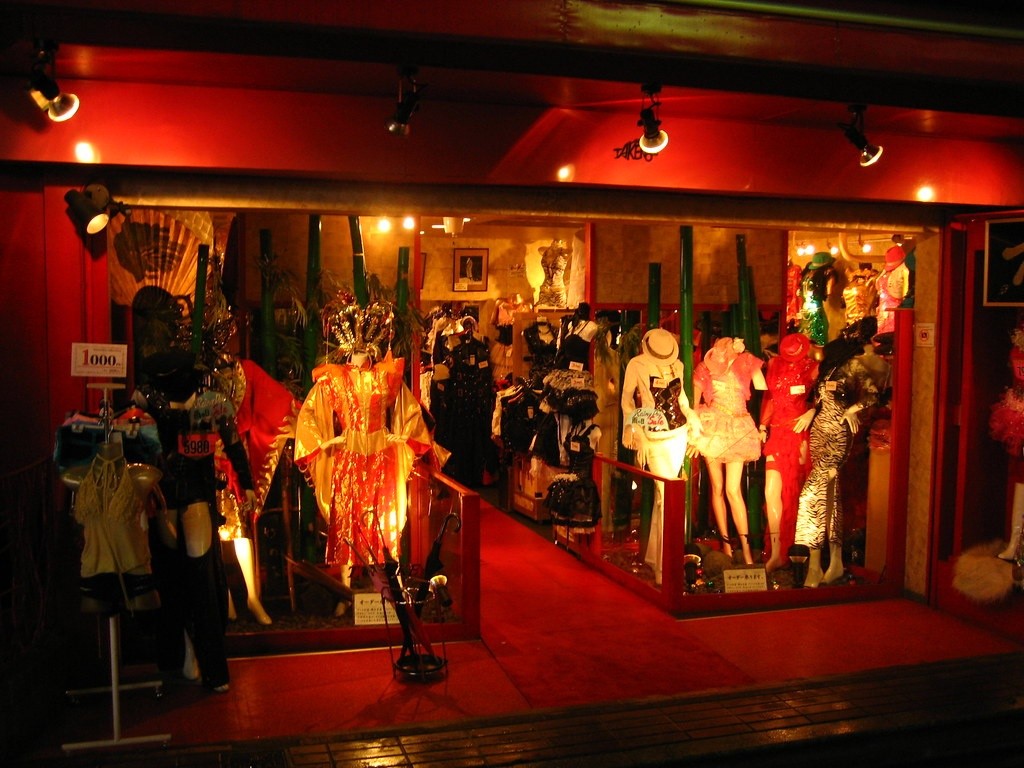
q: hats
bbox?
[886,246,906,262]
[642,328,679,366]
[779,333,810,363]
[808,252,835,269]
[704,337,738,377]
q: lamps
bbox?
[838,100,882,166]
[638,82,668,155]
[65,184,111,235]
[384,66,432,135]
[22,46,81,125]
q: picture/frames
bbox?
[452,248,488,292]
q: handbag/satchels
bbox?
[543,477,602,527]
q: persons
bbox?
[421,246,902,590]
[990,328,1024,563]
[58,307,431,696]
